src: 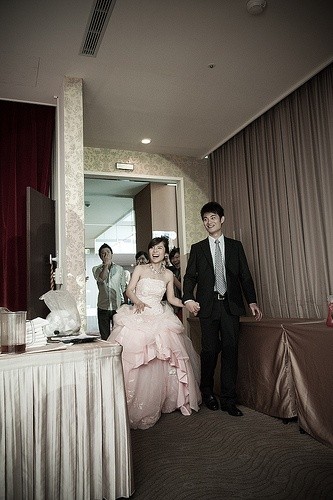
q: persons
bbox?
[182,202,263,416]
[92,242,181,341]
[106,237,202,429]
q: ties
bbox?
[215,240,226,296]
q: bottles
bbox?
[326,294,333,327]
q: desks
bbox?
[0,339,135,500]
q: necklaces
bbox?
[149,263,166,279]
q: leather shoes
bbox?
[202,396,218,411]
[221,404,243,417]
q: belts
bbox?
[215,293,227,301]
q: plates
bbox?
[48,335,100,344]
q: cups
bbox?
[0,311,27,354]
